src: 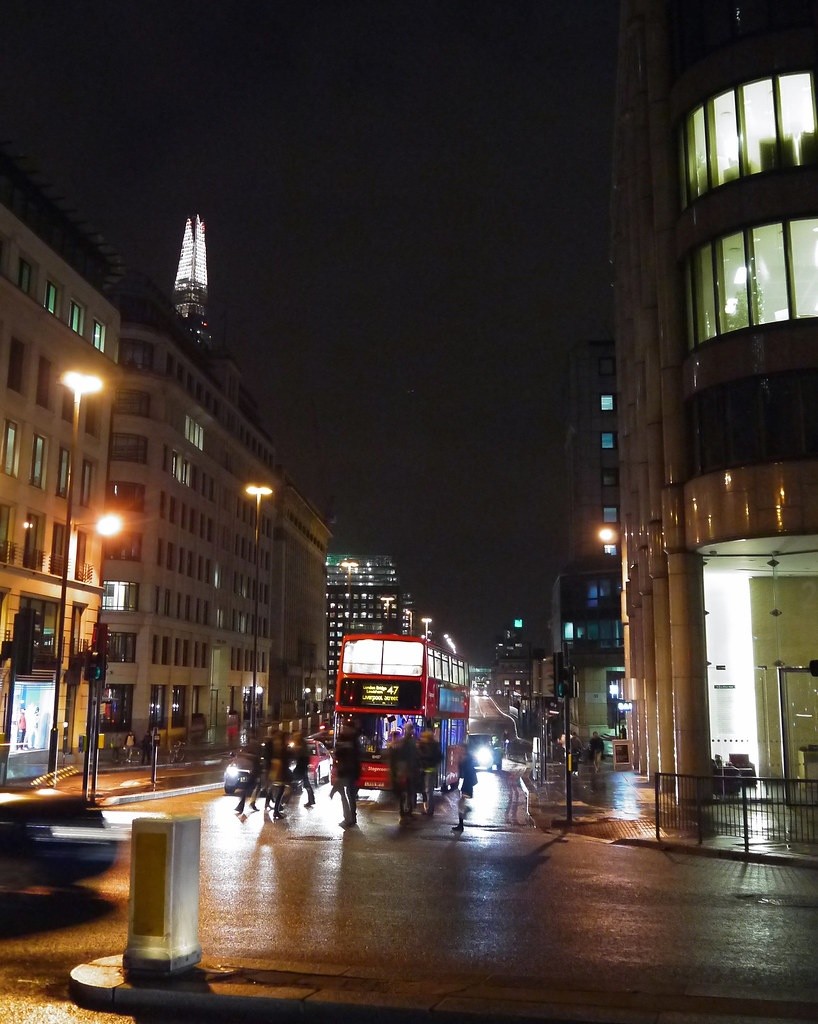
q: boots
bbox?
[452,813,464,832]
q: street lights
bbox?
[599,527,640,771]
[40,371,108,776]
[339,559,361,627]
[405,609,458,654]
[380,596,396,631]
[242,484,274,736]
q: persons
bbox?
[589,731,605,774]
[139,730,152,765]
[123,730,136,762]
[502,728,512,752]
[234,728,318,820]
[15,706,40,752]
[570,732,582,776]
[329,721,479,833]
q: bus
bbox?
[331,631,472,801]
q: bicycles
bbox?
[170,741,185,763]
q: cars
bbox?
[304,740,333,788]
[465,732,503,772]
[224,738,268,795]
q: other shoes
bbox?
[422,811,434,816]
[304,799,315,807]
[339,816,357,826]
[273,811,284,820]
[399,816,408,824]
[250,801,260,811]
[265,803,274,810]
